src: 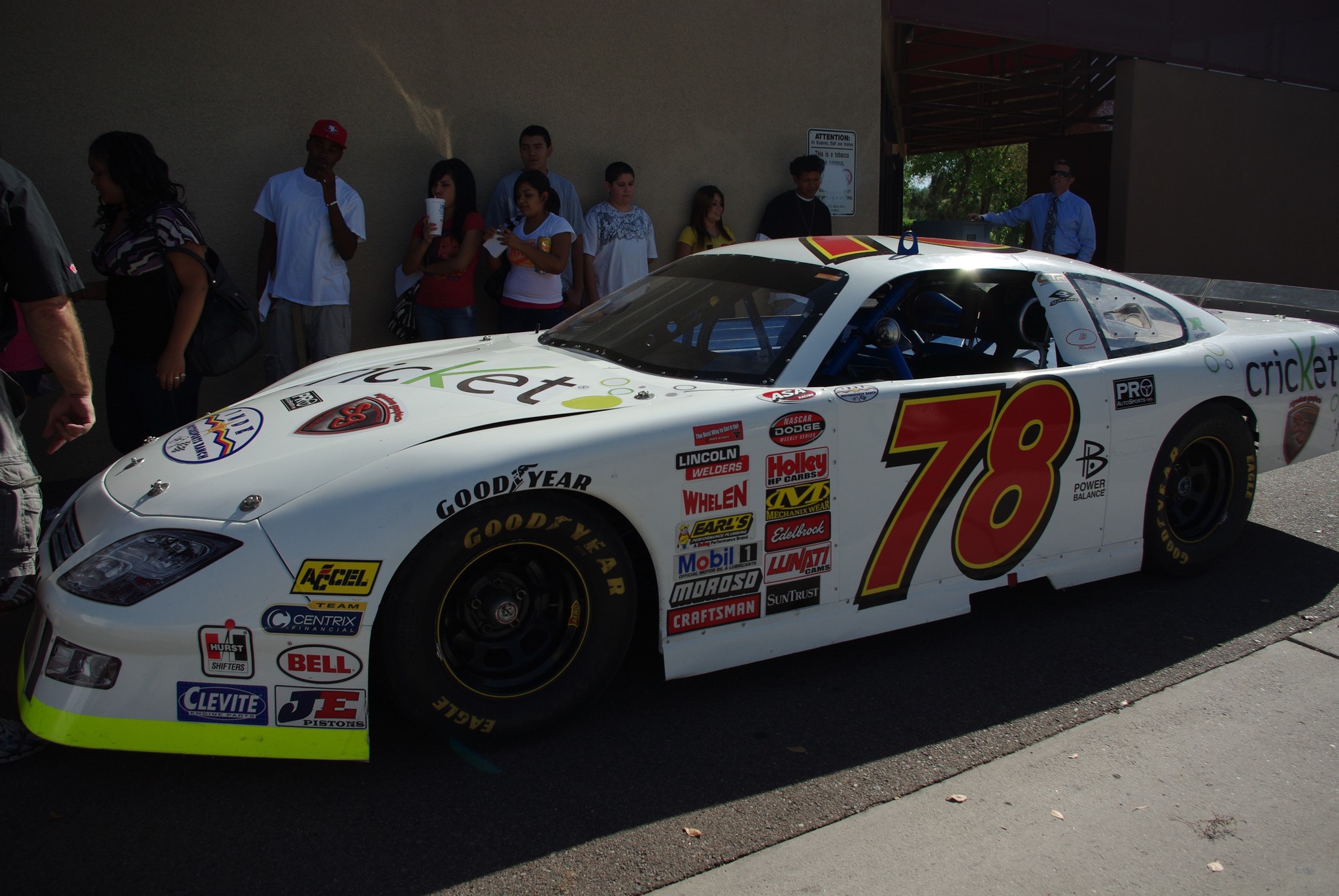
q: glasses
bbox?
[1051,170,1070,178]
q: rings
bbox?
[180,373,186,376]
[174,377,180,379]
[323,179,326,183]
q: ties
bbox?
[1042,197,1057,253]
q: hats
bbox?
[1056,157,1078,172]
[310,120,348,149]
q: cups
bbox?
[425,198,445,237]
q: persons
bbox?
[66,131,211,457]
[583,162,658,303]
[480,173,576,331]
[252,120,365,387]
[756,156,833,315]
[0,156,96,614]
[403,159,482,340]
[486,126,583,333]
[678,186,738,308]
[965,163,1096,262]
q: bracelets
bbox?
[326,201,337,206]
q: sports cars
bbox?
[11,228,1339,782]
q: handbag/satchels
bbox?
[386,287,418,341]
[163,245,259,376]
